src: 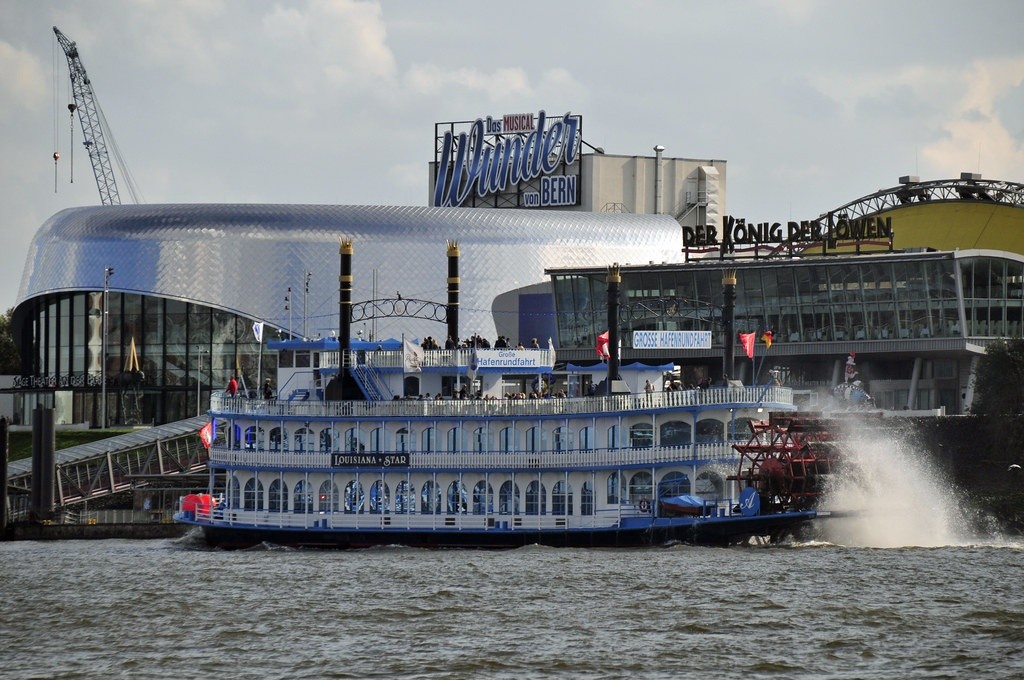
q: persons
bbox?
[721,374,729,402]
[414,333,544,366]
[666,378,678,404]
[584,376,614,398]
[644,379,654,408]
[687,377,712,404]
[259,377,279,401]
[225,375,238,399]
[390,381,579,417]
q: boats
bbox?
[173,333,884,535]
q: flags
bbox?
[252,321,264,342]
[199,423,215,448]
[738,332,756,359]
[760,331,773,349]
